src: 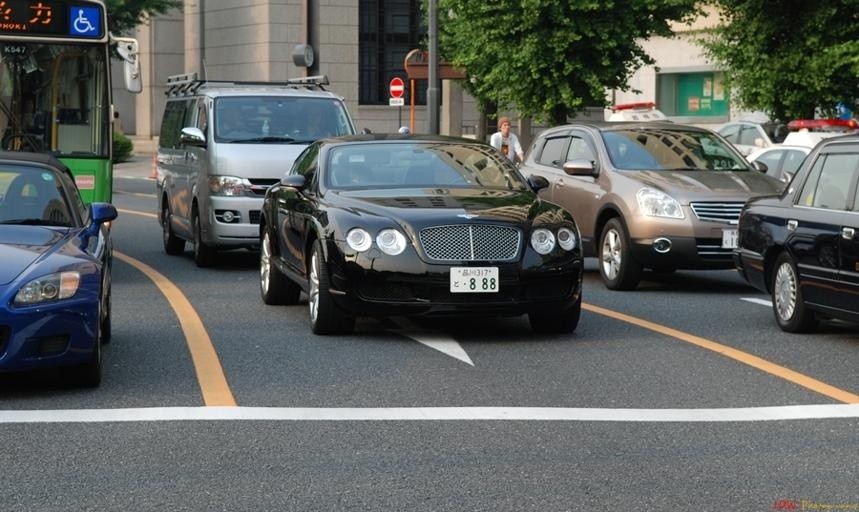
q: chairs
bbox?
[2,175,59,222]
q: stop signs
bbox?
[389,77,405,98]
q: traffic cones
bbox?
[149,153,158,179]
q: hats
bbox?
[497,116,510,130]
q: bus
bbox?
[0,0,143,233]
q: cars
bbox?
[709,113,859,187]
[729,133,859,334]
[258,131,585,335]
[516,119,788,292]
[0,151,118,389]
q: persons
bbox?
[297,103,333,138]
[223,101,245,134]
[490,117,524,163]
[399,126,411,134]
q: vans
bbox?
[155,72,374,266]
